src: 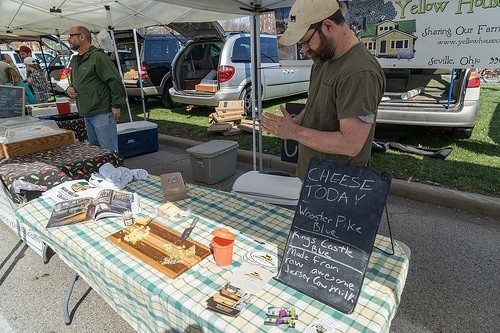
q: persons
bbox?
[259,0,386,182]
[65,26,126,153]
[0,46,49,104]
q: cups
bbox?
[210,227,235,266]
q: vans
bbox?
[0,50,65,81]
[166,21,312,116]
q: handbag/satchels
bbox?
[97,28,114,53]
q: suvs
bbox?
[108,30,187,108]
[376,67,481,139]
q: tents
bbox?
[0,0,298,170]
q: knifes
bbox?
[181,217,199,242]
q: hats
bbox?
[24,57,37,64]
[278,0,339,47]
[20,46,30,52]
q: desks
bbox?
[26,102,78,116]
[14,174,409,333]
[0,117,125,264]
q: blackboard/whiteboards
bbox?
[0,85,25,117]
[273,156,393,314]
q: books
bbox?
[45,188,139,228]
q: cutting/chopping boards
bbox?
[106,219,212,279]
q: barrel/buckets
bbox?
[56,103,70,114]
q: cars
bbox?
[40,35,75,93]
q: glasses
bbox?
[68,34,81,39]
[296,18,333,48]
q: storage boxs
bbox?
[231,171,301,211]
[161,172,187,202]
[56,97,71,114]
[116,121,158,159]
[186,140,239,184]
